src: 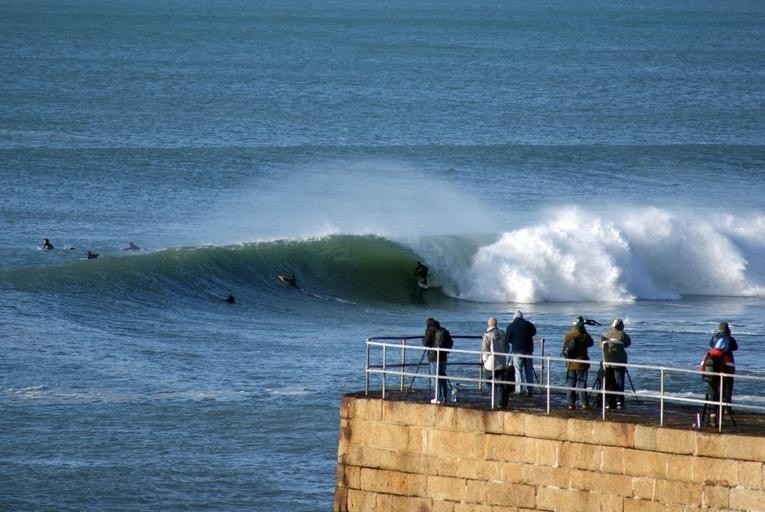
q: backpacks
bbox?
[702,352,724,383]
[561,335,580,357]
[429,326,454,352]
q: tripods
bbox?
[507,349,544,396]
[587,366,641,406]
[698,381,738,427]
[406,350,453,402]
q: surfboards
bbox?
[277,272,297,288]
[417,276,429,289]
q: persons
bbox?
[42,239,54,249]
[478,315,511,410]
[414,262,431,290]
[282,271,301,291]
[87,251,100,260]
[420,319,454,407]
[560,317,595,409]
[705,321,738,427]
[225,295,238,304]
[601,320,632,410]
[505,309,540,399]
[121,241,148,252]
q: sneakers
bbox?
[568,404,574,410]
[604,404,611,411]
[615,402,626,410]
[430,398,446,404]
[582,403,591,409]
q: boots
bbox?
[711,412,718,429]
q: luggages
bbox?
[504,350,516,393]
